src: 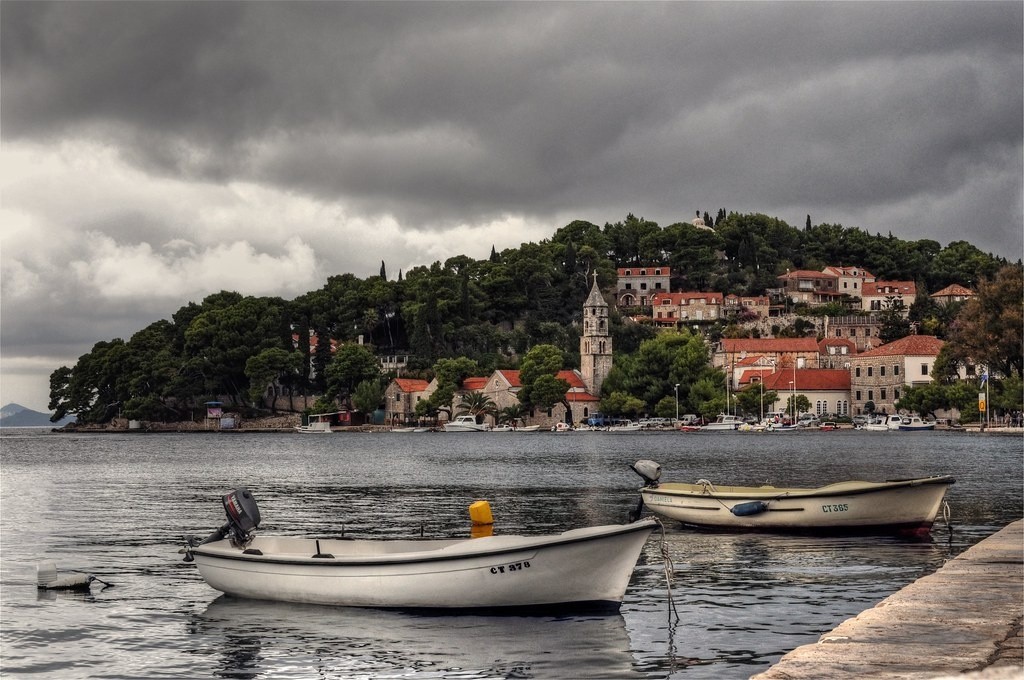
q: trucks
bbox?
[588,412,618,425]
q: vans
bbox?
[765,412,784,423]
[679,414,697,426]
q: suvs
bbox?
[852,414,875,425]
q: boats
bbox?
[176,484,662,608]
[413,427,431,432]
[681,426,701,431]
[768,424,798,431]
[820,422,841,431]
[856,415,936,432]
[441,416,489,432]
[514,425,541,431]
[707,416,743,431]
[491,424,513,431]
[738,424,766,432]
[389,427,414,433]
[933,418,965,432]
[631,456,959,529]
[292,422,335,434]
[610,420,641,432]
[551,423,571,431]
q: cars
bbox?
[797,416,822,427]
[639,417,678,427]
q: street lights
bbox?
[726,365,730,414]
[789,380,793,426]
[675,383,681,420]
